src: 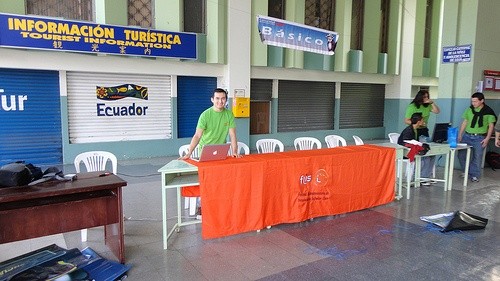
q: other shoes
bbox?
[460,173,465,178]
[471,177,479,184]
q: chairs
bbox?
[179,135,365,214]
[74,151,118,241]
[388,132,415,181]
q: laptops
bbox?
[426,122,452,141]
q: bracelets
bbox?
[431,102,434,104]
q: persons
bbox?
[397,90,441,186]
[457,92,496,183]
[495,114,500,148]
[178,88,243,216]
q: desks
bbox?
[0,170,128,264]
[442,143,474,190]
[400,143,451,200]
[157,144,404,249]
